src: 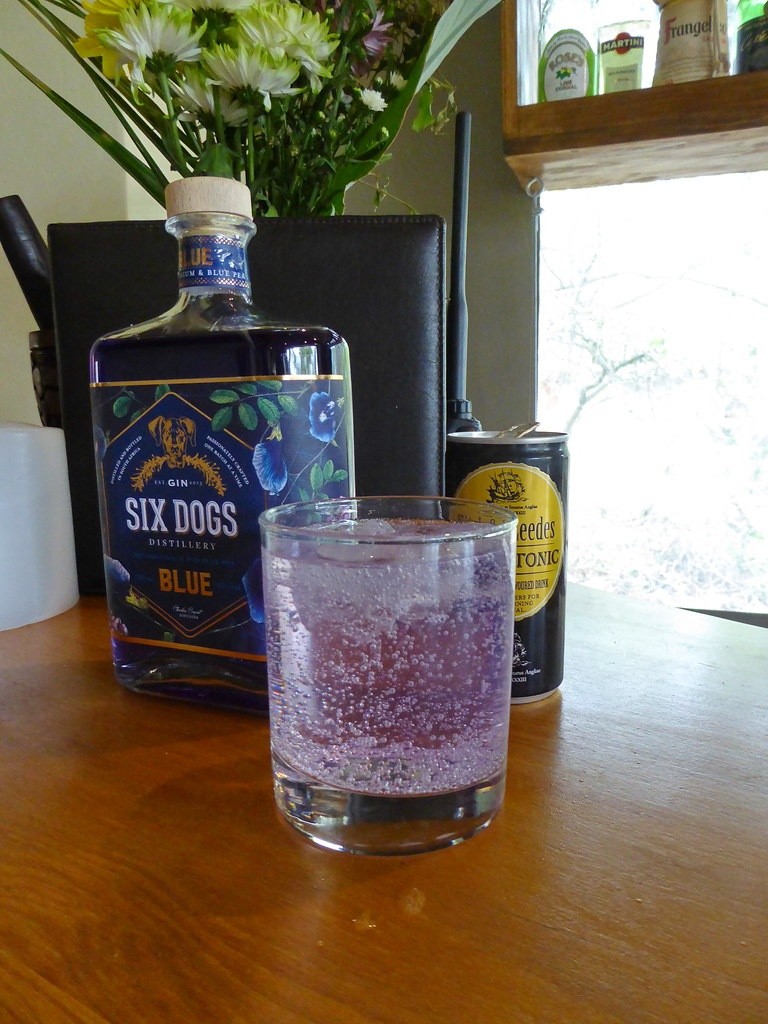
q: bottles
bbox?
[89,173,357,715]
[736,0,768,72]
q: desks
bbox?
[0,580,768,1024]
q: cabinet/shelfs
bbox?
[502,0,768,194]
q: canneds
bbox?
[443,423,571,707]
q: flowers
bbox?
[11,0,517,219]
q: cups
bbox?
[597,20,652,93]
[260,494,519,855]
